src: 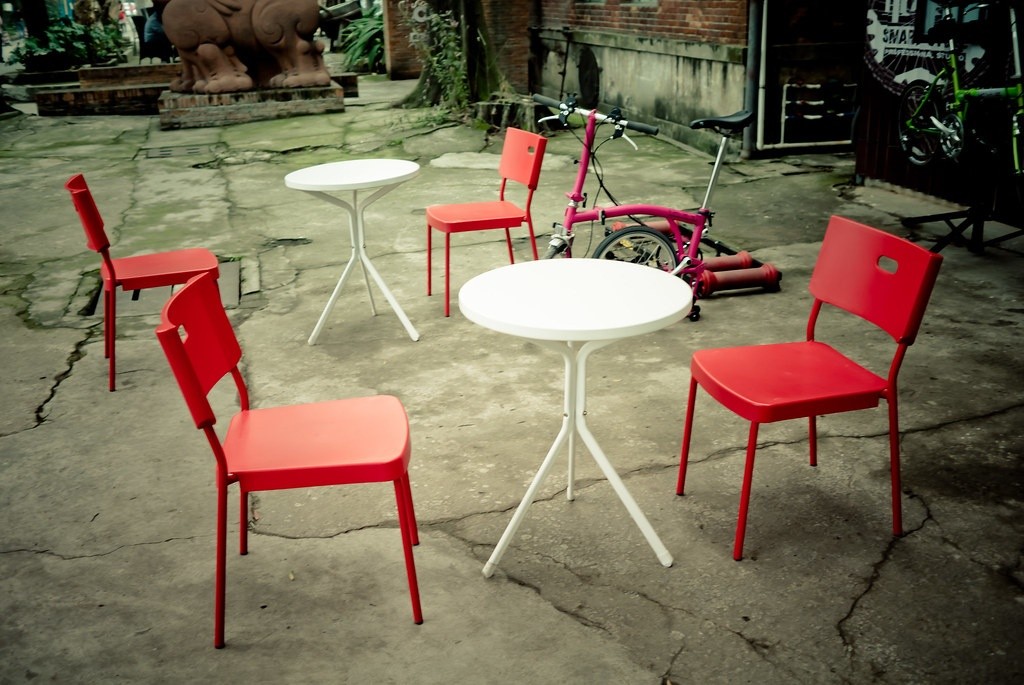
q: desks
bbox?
[284,158,421,346]
[457,257,694,579]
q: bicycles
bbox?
[895,0,1024,177]
[533,94,752,322]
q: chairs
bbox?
[131,16,166,63]
[140,7,155,16]
[424,127,547,318]
[154,270,423,650]
[675,216,944,560]
[64,173,221,392]
